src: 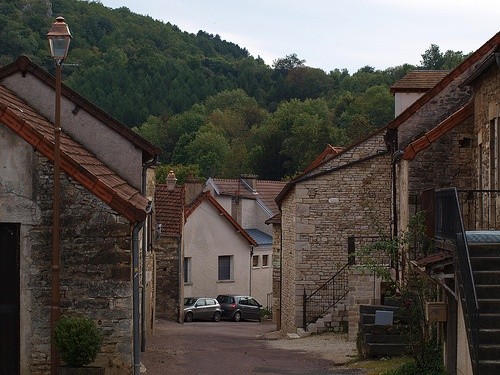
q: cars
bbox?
[182,297,221,323]
[217,295,269,322]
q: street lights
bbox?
[45,15,75,375]
[164,170,175,238]
